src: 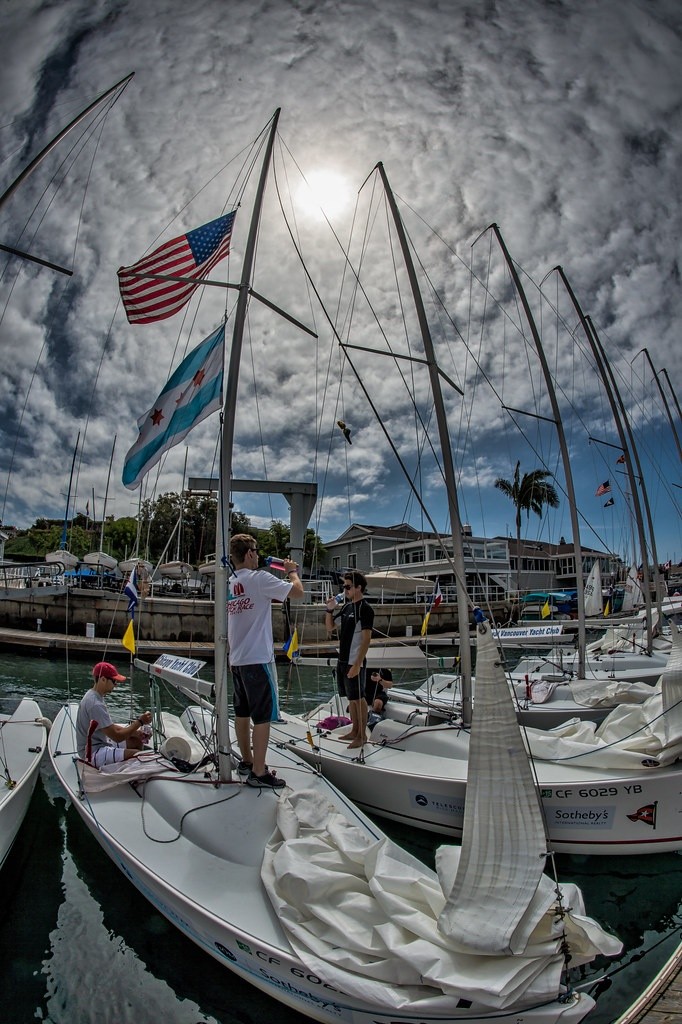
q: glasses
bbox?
[343,584,351,590]
[109,678,116,684]
[251,549,258,555]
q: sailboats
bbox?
[0,69,681,1024]
[40,102,625,1023]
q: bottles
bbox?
[327,592,347,610]
[264,556,300,573]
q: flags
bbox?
[121,321,225,492]
[115,209,237,325]
[662,560,671,571]
[282,627,300,663]
[604,498,614,508]
[595,480,611,497]
[617,455,624,464]
[120,620,135,656]
[541,601,550,619]
[603,600,610,616]
[638,563,643,582]
[420,611,431,637]
[432,580,443,607]
[124,565,139,615]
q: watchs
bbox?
[326,609,334,613]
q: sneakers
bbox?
[238,762,268,775]
[246,770,286,788]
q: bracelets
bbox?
[137,719,144,727]
[288,569,297,576]
[378,679,382,683]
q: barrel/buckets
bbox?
[159,736,191,764]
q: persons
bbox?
[225,533,304,790]
[673,588,680,596]
[77,661,155,769]
[364,667,393,717]
[325,572,374,750]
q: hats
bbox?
[93,662,126,682]
